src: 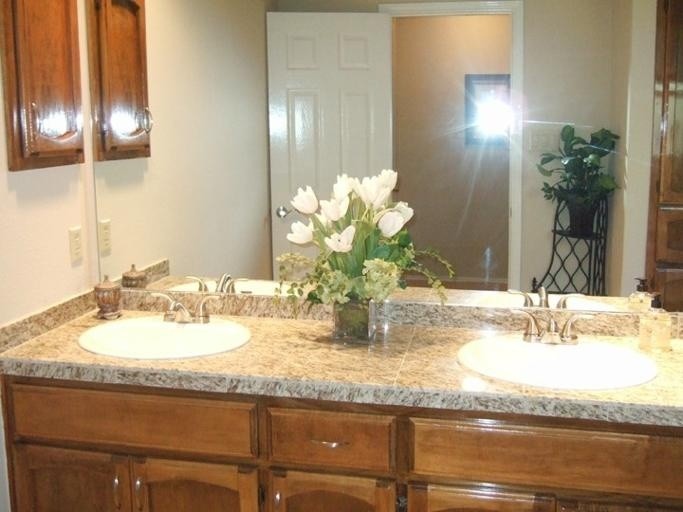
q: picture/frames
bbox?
[464,73,510,147]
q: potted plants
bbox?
[536,125,620,235]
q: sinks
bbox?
[480,292,603,309]
[79,316,252,359]
[169,278,288,293]
[456,332,660,392]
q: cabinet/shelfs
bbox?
[85,1,151,162]
[0,1,84,172]
[404,406,681,512]
[0,375,263,511]
[262,394,402,511]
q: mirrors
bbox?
[82,0,682,316]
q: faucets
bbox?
[215,273,234,292]
[168,301,193,320]
[538,285,551,307]
[537,317,561,346]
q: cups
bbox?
[367,295,404,356]
[480,246,498,290]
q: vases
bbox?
[333,299,378,343]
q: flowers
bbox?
[275,169,456,336]
